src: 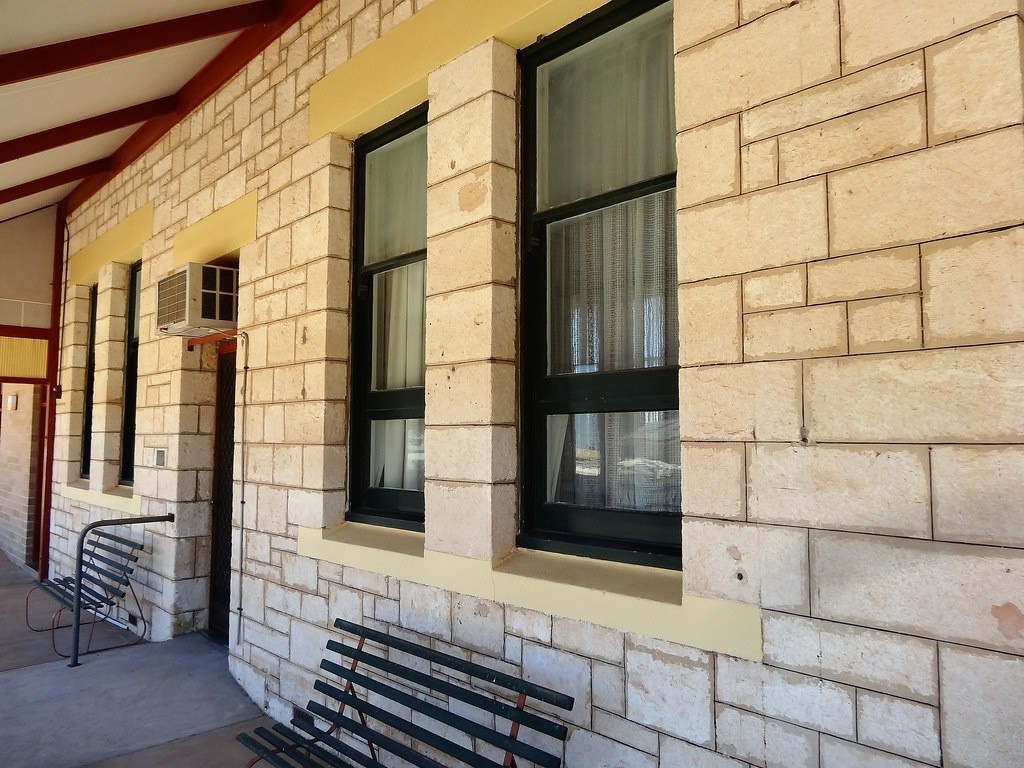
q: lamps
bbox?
[7,395,17,410]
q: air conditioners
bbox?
[154,261,239,340]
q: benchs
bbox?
[24,530,147,659]
[235,617,575,768]
